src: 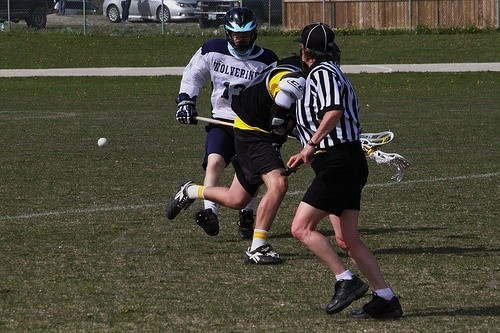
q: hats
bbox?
[293,23,335,52]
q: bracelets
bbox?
[308,140,319,147]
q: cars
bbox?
[53,0,99,16]
[1,0,52,28]
[196,0,244,28]
[102,0,199,23]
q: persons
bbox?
[289,22,405,320]
[121,0,133,24]
[175,6,282,241]
[169,42,340,264]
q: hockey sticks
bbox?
[362,147,412,182]
[192,115,395,152]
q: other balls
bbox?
[97,137,109,148]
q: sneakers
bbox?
[237,209,256,241]
[244,244,285,265]
[325,274,369,314]
[195,209,219,236]
[167,179,196,220]
[346,291,403,318]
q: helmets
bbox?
[225,7,259,60]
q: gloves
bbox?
[176,93,198,125]
